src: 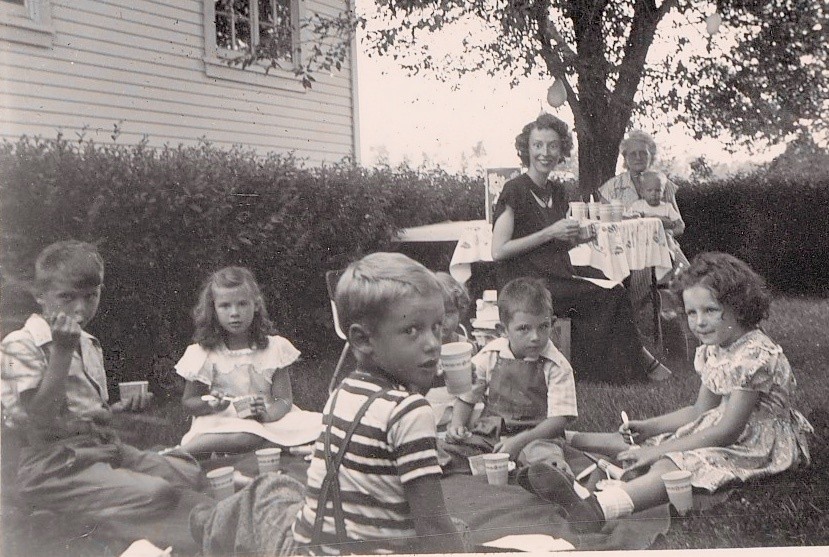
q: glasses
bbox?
[625,151,650,159]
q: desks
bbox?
[392,219,672,355]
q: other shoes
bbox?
[530,463,605,532]
[31,510,58,536]
[516,460,539,498]
[646,358,685,389]
[188,503,215,548]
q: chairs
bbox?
[326,269,350,396]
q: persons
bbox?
[491,114,679,384]
[188,252,471,557]
[174,263,324,455]
[0,239,201,521]
[530,249,815,531]
[424,272,486,431]
[436,274,578,495]
[597,129,691,345]
[625,171,684,289]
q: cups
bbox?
[578,224,592,240]
[233,396,256,419]
[206,466,236,500]
[255,447,282,475]
[662,470,693,516]
[118,381,149,401]
[440,342,473,394]
[568,201,624,223]
[483,453,510,485]
[467,455,485,475]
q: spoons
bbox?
[621,410,640,450]
